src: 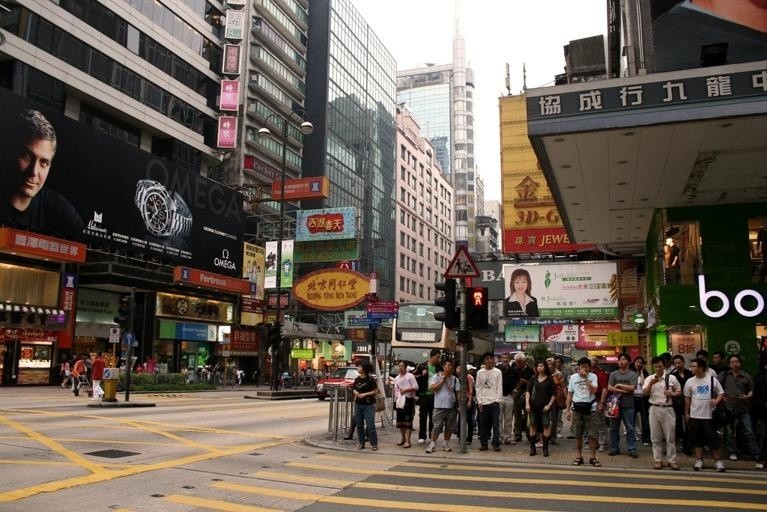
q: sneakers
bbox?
[667,462,679,469]
[478,436,522,451]
[608,448,618,455]
[713,461,724,472]
[442,444,450,451]
[426,445,435,452]
[397,440,411,448]
[636,429,650,447]
[418,439,424,444]
[536,442,543,447]
[629,451,637,458]
[731,455,737,459]
[343,436,352,440]
[358,445,378,451]
[655,462,663,469]
[695,460,704,470]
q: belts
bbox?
[651,403,672,407]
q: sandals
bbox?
[572,457,583,465]
[589,457,600,466]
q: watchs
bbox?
[133,179,193,239]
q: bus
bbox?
[388,303,494,386]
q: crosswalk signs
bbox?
[444,246,481,279]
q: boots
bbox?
[528,434,536,456]
[542,434,549,456]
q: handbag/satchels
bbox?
[672,394,685,414]
[574,401,591,414]
[712,408,727,430]
[604,394,619,417]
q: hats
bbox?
[467,365,476,370]
[407,366,414,372]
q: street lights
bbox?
[256,109,315,391]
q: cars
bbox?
[317,367,360,401]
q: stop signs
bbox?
[110,327,121,344]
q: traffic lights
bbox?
[465,287,489,329]
[434,279,457,330]
[114,296,131,329]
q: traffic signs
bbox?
[348,300,398,326]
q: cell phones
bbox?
[655,375,659,379]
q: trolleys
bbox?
[75,376,93,396]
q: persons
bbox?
[757,224,767,283]
[393,350,767,472]
[61,350,331,401]
[664,246,672,287]
[0,109,85,242]
[505,269,539,317]
[666,238,680,286]
[343,359,378,452]
